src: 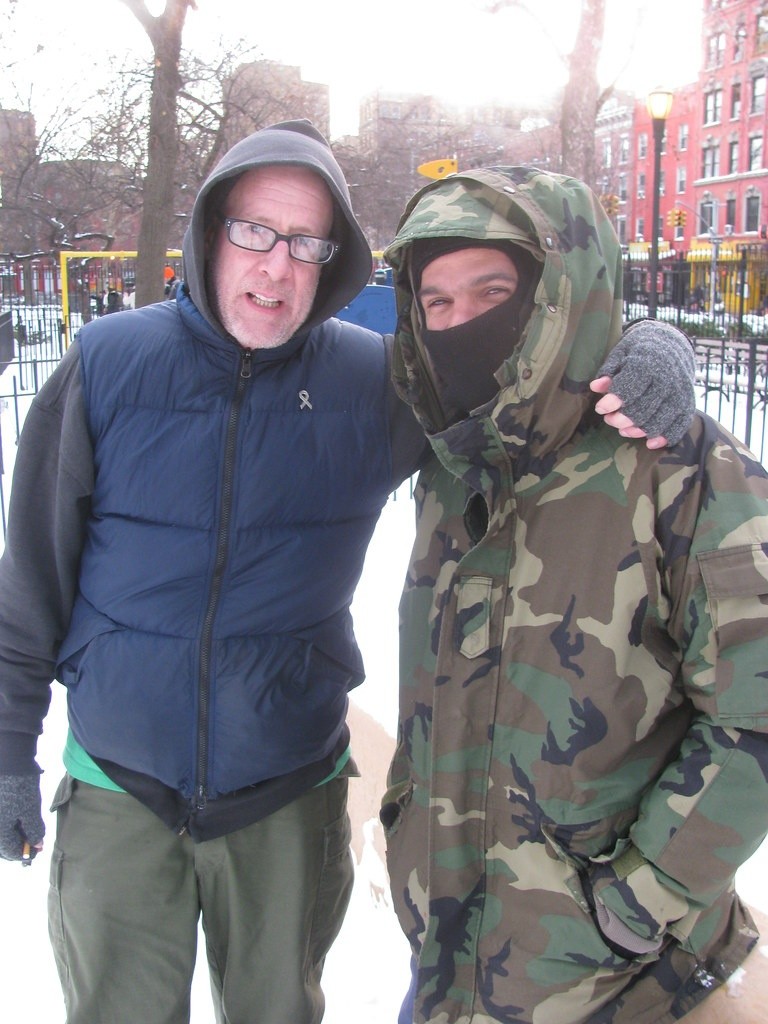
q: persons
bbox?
[378,164,768,1024]
[0,121,696,1024]
[89,266,179,318]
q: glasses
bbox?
[211,206,341,265]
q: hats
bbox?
[410,237,545,411]
